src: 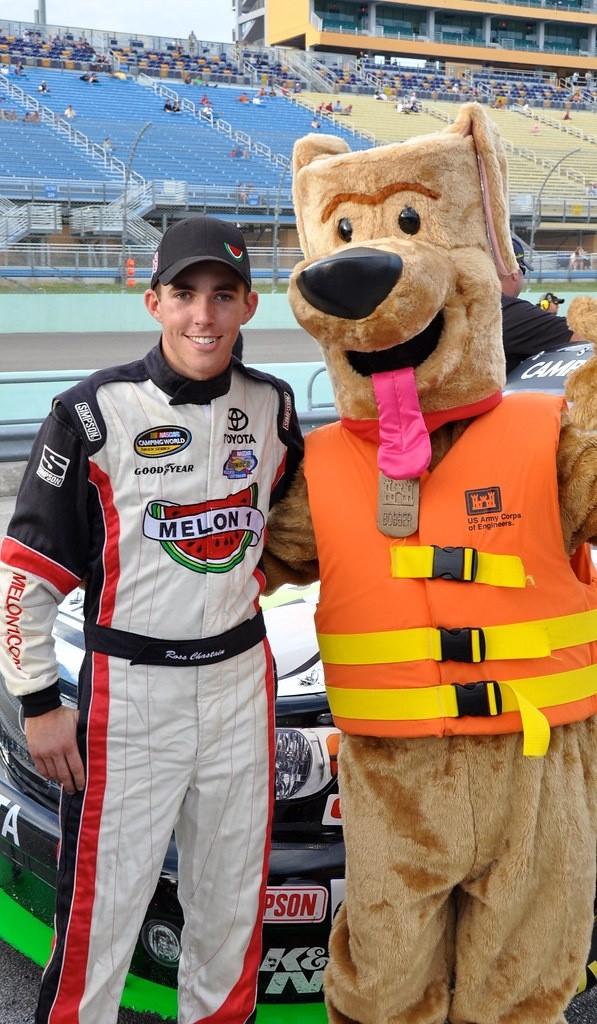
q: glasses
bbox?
[518,266,527,276]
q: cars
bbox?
[0,342,597,1024]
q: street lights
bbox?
[526,146,582,292]
[122,120,154,289]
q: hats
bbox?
[152,218,252,294]
[539,292,564,305]
[512,237,535,271]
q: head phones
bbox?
[541,294,554,311]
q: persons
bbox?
[586,184,597,197]
[0,23,597,162]
[494,238,587,377]
[536,292,565,315]
[1,214,310,1024]
[237,181,264,206]
[570,246,592,271]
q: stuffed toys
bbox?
[254,97,597,1024]
